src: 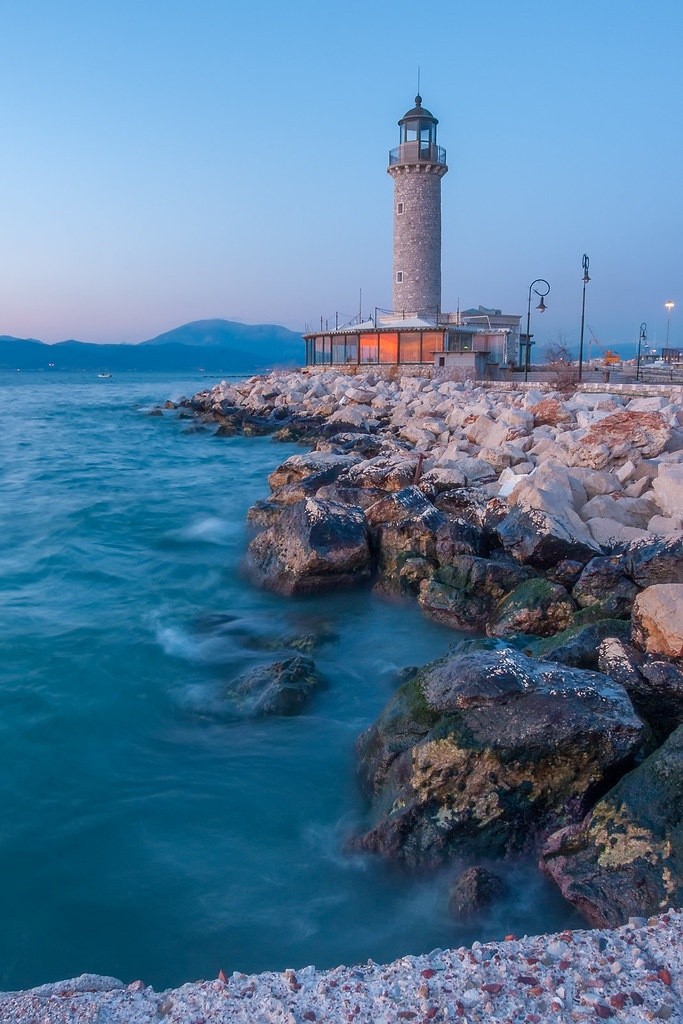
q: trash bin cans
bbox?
[486,362,499,380]
[499,364,511,381]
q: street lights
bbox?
[523,278,551,382]
[578,253,590,382]
[635,322,648,381]
[664,300,675,363]
